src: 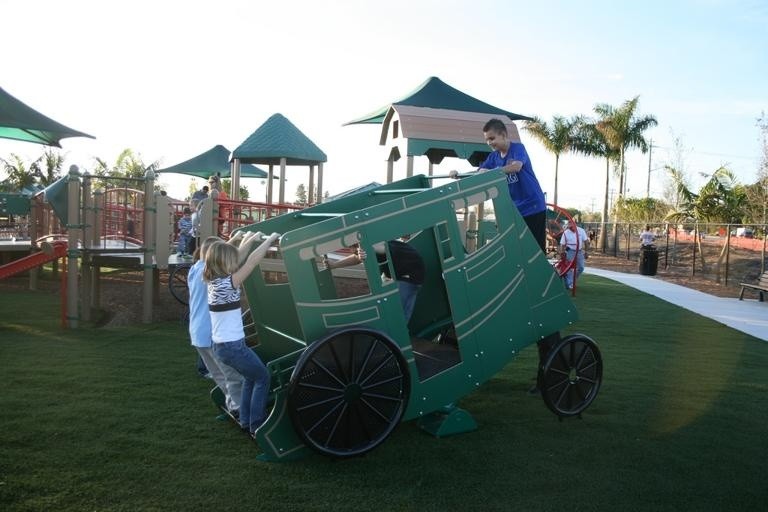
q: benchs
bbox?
[738,269,768,301]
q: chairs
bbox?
[381,240,425,325]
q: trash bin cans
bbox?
[638,244,659,276]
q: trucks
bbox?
[735,227,753,240]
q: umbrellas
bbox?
[154,145,279,179]
[341,77,534,187]
[0,86,96,148]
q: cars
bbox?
[730,230,735,237]
[689,230,704,239]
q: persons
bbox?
[450,119,547,256]
[560,221,589,289]
[324,239,425,326]
[639,225,655,246]
[160,172,280,435]
